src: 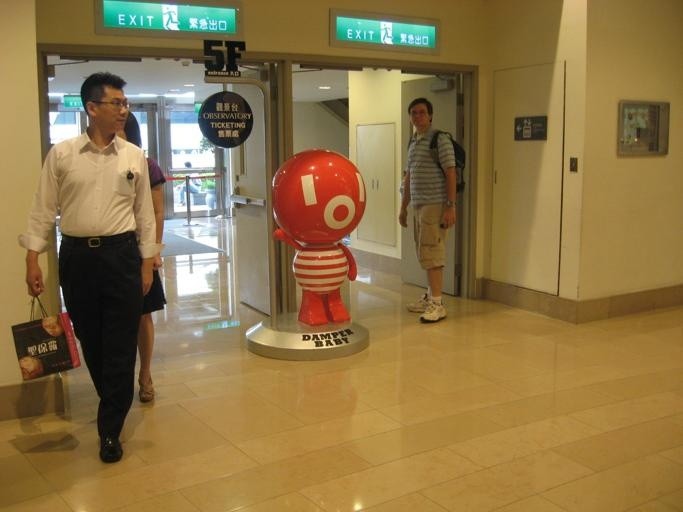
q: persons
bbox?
[174,162,201,207]
[204,166,227,208]
[398,96,457,323]
[19,73,166,462]
[119,109,165,405]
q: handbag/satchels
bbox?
[11,312,81,381]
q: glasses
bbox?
[92,100,130,111]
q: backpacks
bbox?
[430,131,465,193]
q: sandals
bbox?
[137,375,154,401]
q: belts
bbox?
[61,231,135,248]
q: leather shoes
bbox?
[99,431,123,463]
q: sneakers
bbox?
[419,303,447,322]
[406,295,431,312]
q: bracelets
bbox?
[444,200,457,208]
